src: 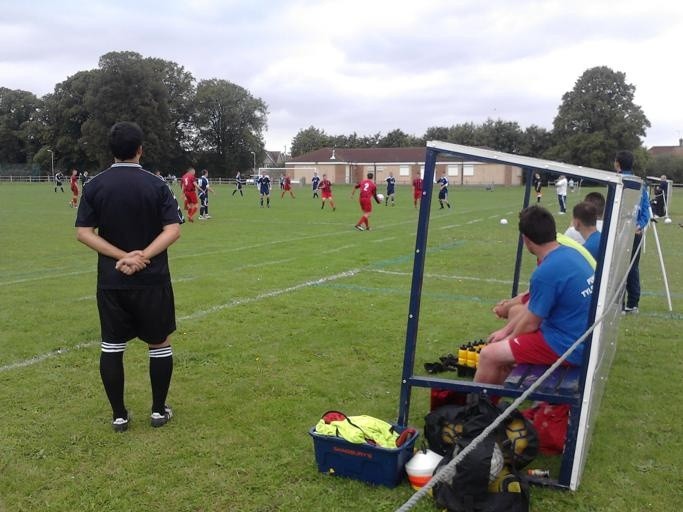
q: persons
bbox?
[154,170,164,181]
[534,172,544,202]
[69,170,79,209]
[572,202,601,259]
[649,185,666,217]
[563,192,604,245]
[54,170,64,192]
[568,178,574,192]
[467,206,595,407]
[81,171,89,190]
[182,167,450,231]
[553,175,568,214]
[76,121,182,431]
[613,151,651,316]
[490,204,597,321]
[654,175,667,201]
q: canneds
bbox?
[527,467,551,479]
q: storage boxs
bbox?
[308,422,419,490]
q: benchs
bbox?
[501,361,587,485]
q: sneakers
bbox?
[150,406,173,427]
[625,307,638,313]
[355,225,364,230]
[112,413,130,432]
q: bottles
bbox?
[457,338,488,369]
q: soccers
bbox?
[501,218,508,224]
[665,218,672,224]
[377,194,384,201]
[425,402,539,511]
